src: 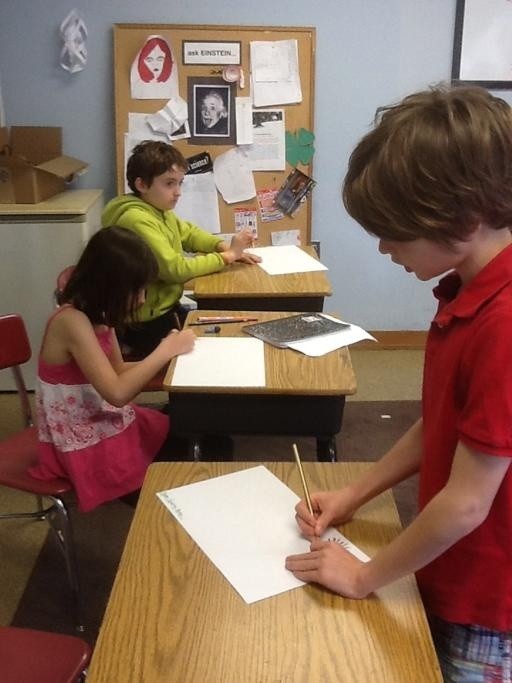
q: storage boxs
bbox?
[0,126,89,204]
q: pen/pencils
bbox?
[188,316,258,326]
[293,443,320,541]
[174,312,182,331]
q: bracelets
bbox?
[220,252,230,265]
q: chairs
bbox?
[55,265,171,391]
[0,626,92,683]
[0,313,83,631]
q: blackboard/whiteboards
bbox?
[451,0,512,90]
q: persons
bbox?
[35,224,196,513]
[137,36,172,82]
[101,138,262,358]
[284,80,511,683]
[194,84,227,133]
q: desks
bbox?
[162,310,358,462]
[84,462,445,683]
[194,246,333,312]
